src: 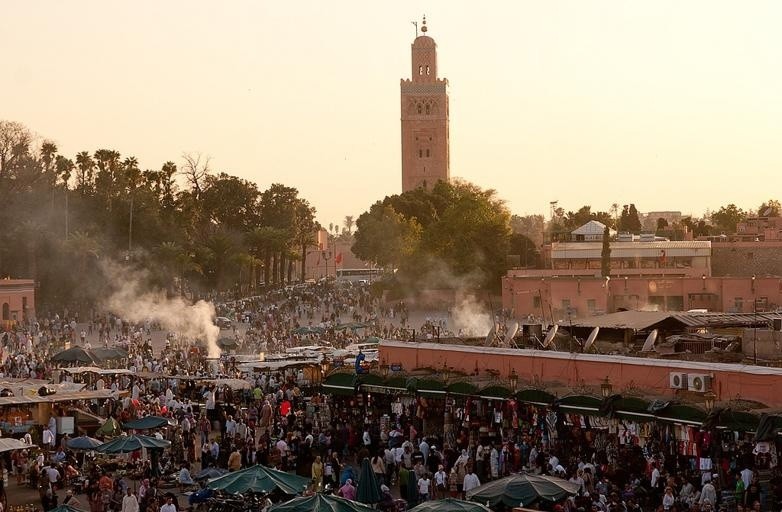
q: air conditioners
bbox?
[670,372,688,390]
[688,374,712,393]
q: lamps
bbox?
[541,278,551,288]
[576,277,582,293]
[702,274,707,289]
[623,276,628,291]
[751,275,755,290]
